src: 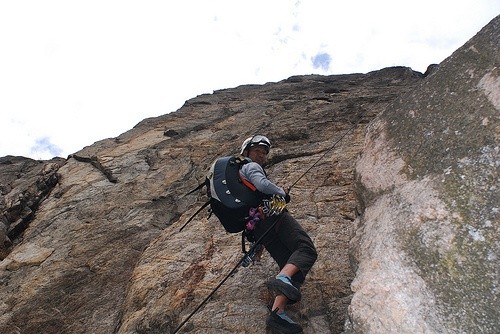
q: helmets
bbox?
[239,135,271,155]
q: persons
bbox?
[236,135,317,334]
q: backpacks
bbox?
[205,156,266,234]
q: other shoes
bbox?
[267,305,304,333]
[272,274,301,301]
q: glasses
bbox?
[251,135,271,147]
